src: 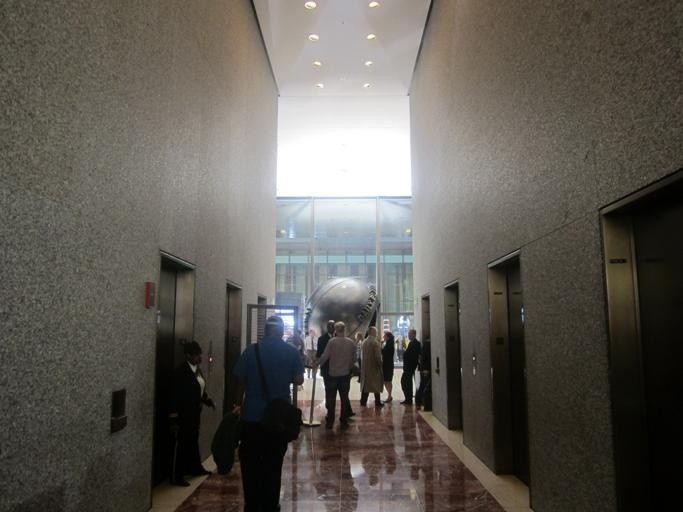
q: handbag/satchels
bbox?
[252,341,303,442]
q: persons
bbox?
[399,329,421,405]
[311,321,354,426]
[305,328,318,379]
[232,316,305,512]
[360,326,384,407]
[168,340,216,487]
[355,332,363,383]
[380,332,394,403]
[315,320,356,419]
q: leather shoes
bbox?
[416,404,421,410]
[382,397,392,403]
[400,400,411,404]
[349,412,355,416]
[375,402,384,407]
[424,407,432,412]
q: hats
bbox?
[265,315,285,327]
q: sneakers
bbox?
[340,423,349,430]
[191,467,211,476]
[169,478,191,487]
[326,423,333,429]
